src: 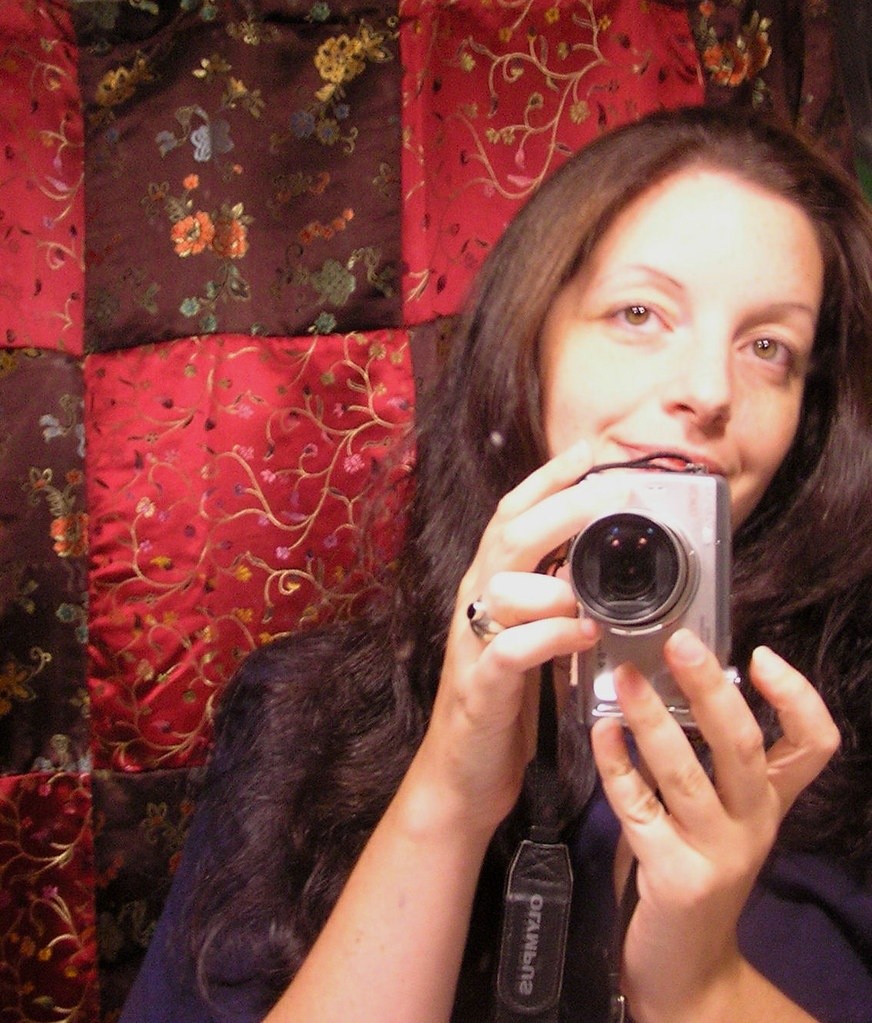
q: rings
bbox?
[466,596,503,643]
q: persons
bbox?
[116,107,872,1023]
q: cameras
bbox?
[568,466,740,734]
